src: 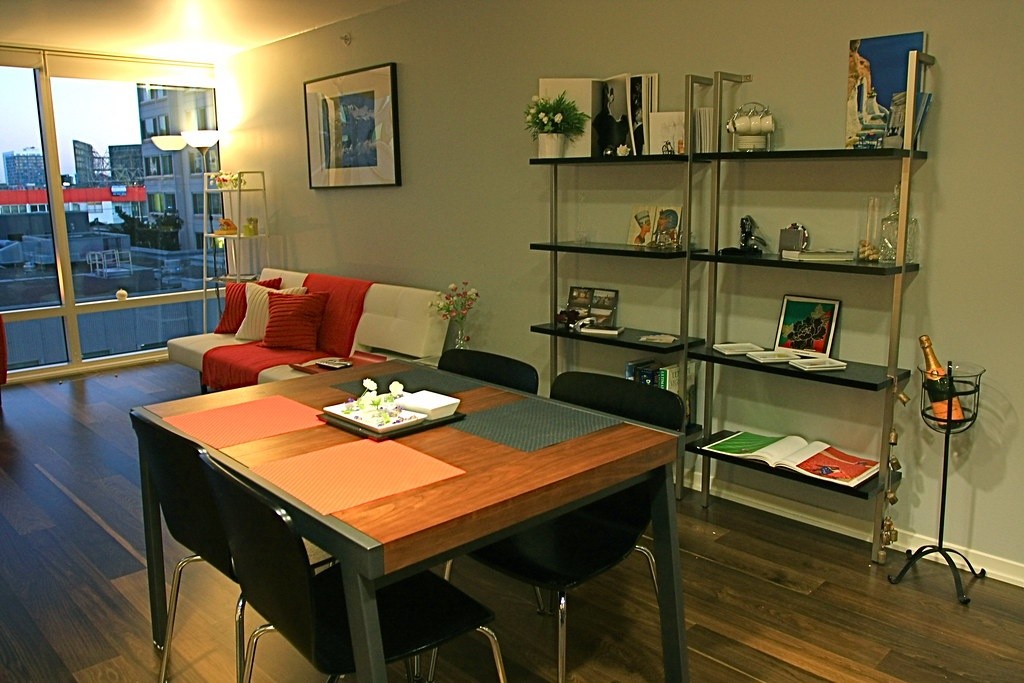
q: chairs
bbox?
[427,370,684,683]
[438,348,539,395]
[128,410,507,683]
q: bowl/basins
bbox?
[395,391,460,421]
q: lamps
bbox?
[180,130,222,325]
[151,135,188,152]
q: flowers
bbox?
[209,168,246,190]
[428,281,480,349]
[524,89,588,146]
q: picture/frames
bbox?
[302,62,402,189]
[567,286,620,328]
[772,293,842,358]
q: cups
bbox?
[726,107,774,132]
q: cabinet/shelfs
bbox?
[526,48,937,565]
[202,170,271,334]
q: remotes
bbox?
[315,359,353,369]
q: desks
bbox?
[132,358,690,683]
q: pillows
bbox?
[213,277,329,351]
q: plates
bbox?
[323,390,426,433]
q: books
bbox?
[782,249,854,261]
[625,357,696,428]
[581,325,625,335]
[700,431,881,488]
[538,73,714,158]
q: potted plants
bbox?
[178,264,226,291]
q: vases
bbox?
[454,327,469,350]
[537,133,566,159]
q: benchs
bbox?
[167,268,456,395]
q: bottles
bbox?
[878,185,919,264]
[917,333,967,430]
